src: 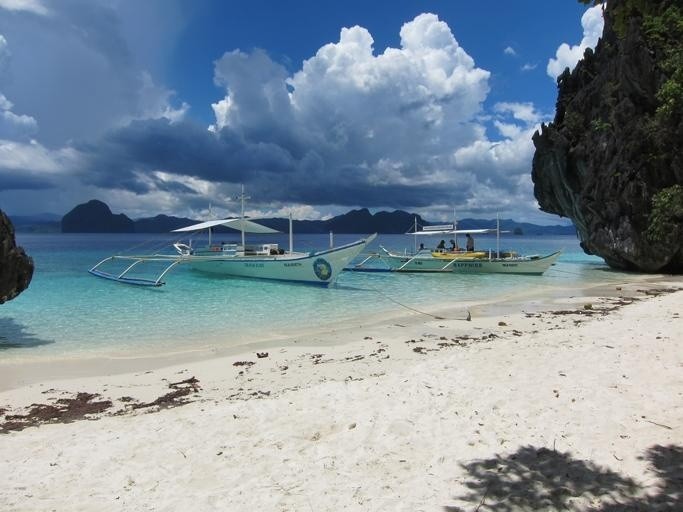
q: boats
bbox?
[341,208,563,278]
[86,184,377,289]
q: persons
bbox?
[464,233,474,251]
[436,239,446,250]
[418,243,424,252]
[448,239,458,250]
